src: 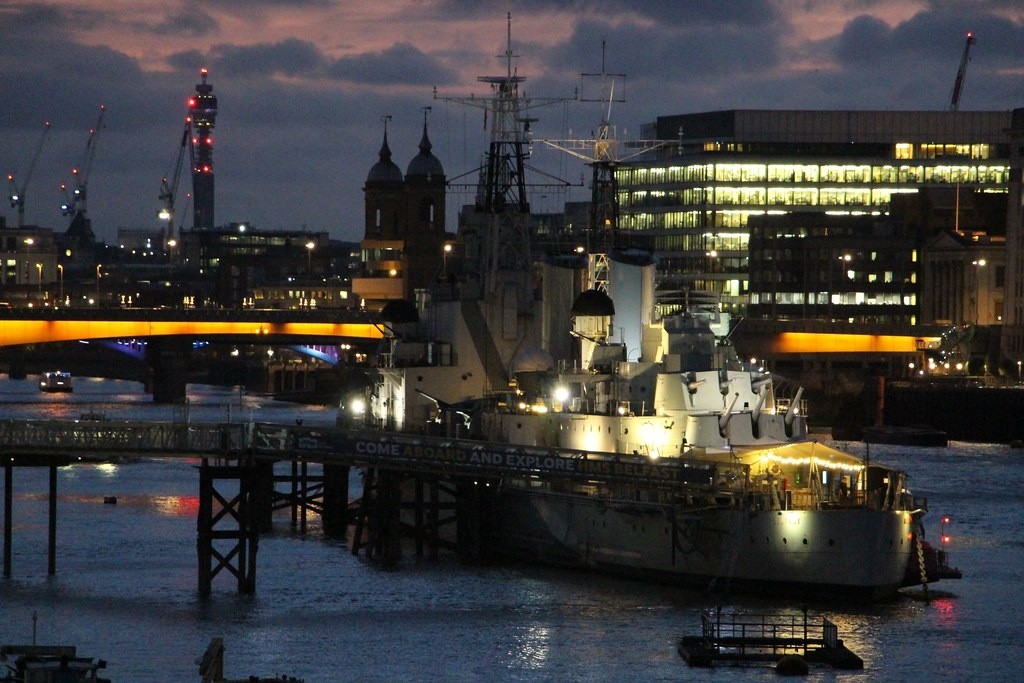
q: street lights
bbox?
[36,263,43,307]
[96,263,103,304]
[57,265,64,306]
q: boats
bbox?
[341,254,965,607]
[0,612,306,683]
[39,371,73,392]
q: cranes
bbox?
[5,119,51,226]
[55,104,107,230]
[159,66,218,249]
[940,32,977,112]
